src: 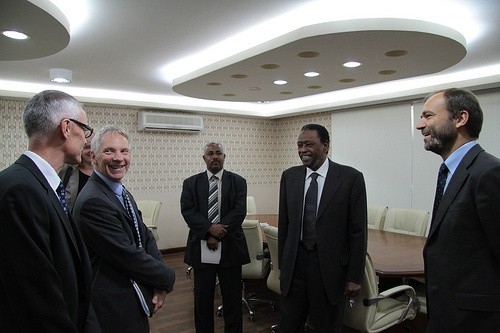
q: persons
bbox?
[0,88,176,333]
[277,125,368,333]
[180,142,251,333]
[417,88,500,333]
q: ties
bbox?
[430,163,449,235]
[122,187,142,247]
[208,174,220,225]
[301,171,319,254]
[56,180,70,220]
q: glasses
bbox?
[70,118,94,138]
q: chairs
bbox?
[187,196,432,333]
[136,199,162,241]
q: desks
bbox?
[243,214,428,286]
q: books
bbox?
[133,279,156,317]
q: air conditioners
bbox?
[137,111,204,133]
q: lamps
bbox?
[49,68,73,84]
